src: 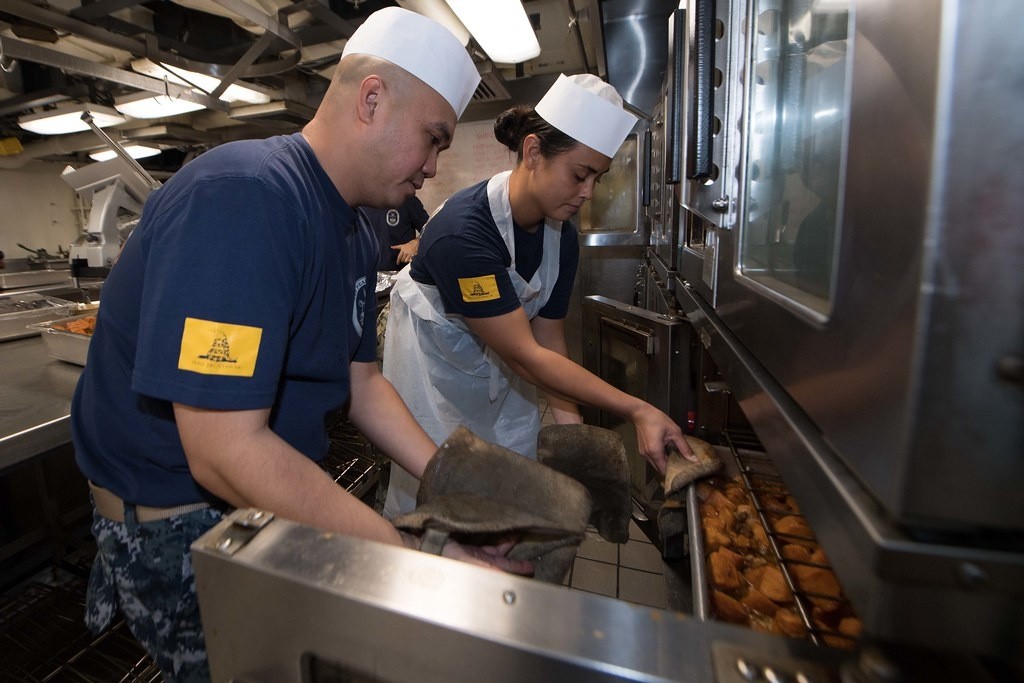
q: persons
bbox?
[70,6,534,683]
[373,72,700,522]
[357,195,431,273]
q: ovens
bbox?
[193,0,1024,683]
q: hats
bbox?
[338,6,482,122]
[535,73,639,159]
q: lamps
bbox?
[395,0,542,64]
[88,142,163,162]
[17,102,126,135]
[114,57,270,120]
